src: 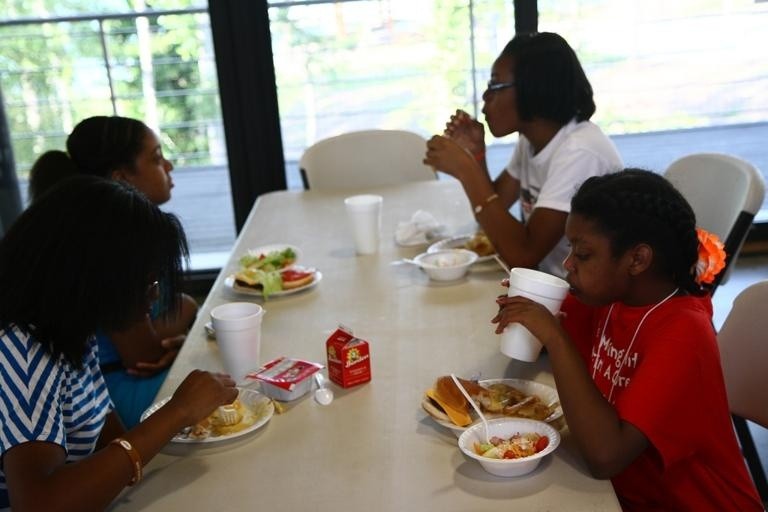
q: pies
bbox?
[234,273,315,293]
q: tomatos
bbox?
[503,450,515,458]
[534,436,549,453]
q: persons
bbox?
[24,114,199,432]
[0,172,239,512]
[420,30,629,291]
[489,167,765,511]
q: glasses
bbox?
[486,78,516,92]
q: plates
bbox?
[222,267,323,297]
[425,235,499,264]
[249,245,303,272]
[138,386,276,446]
[430,378,564,440]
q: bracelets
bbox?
[474,148,488,161]
[111,435,142,490]
[471,193,499,216]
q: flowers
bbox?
[689,226,730,285]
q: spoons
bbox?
[312,371,333,406]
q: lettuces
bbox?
[237,247,296,300]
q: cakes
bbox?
[191,398,244,435]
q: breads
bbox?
[464,231,496,256]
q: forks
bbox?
[217,405,238,424]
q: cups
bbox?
[208,301,264,388]
[342,193,383,256]
[499,267,571,364]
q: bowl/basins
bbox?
[412,248,478,283]
[457,418,562,479]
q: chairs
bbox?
[715,281,767,512]
[661,153,767,289]
[299,129,440,189]
